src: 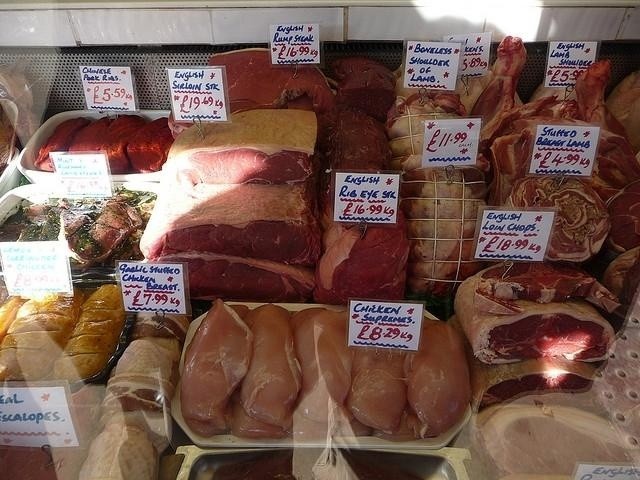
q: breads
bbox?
[0,296,23,339]
[54,284,124,380]
[0,288,84,381]
